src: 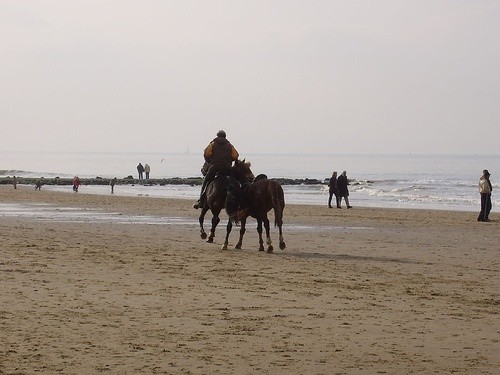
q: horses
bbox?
[198,161,248,250]
[232,157,286,253]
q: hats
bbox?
[218,130,225,135]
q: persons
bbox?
[328,172,341,208]
[479,169,492,221]
[192,130,240,209]
[477,172,492,222]
[137,163,145,179]
[144,164,150,179]
[72,176,80,192]
[336,170,352,209]
[110,177,116,193]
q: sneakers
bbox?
[337,207,342,208]
[192,201,202,209]
[328,207,333,208]
[478,218,483,221]
[347,206,352,209]
[483,219,491,221]
